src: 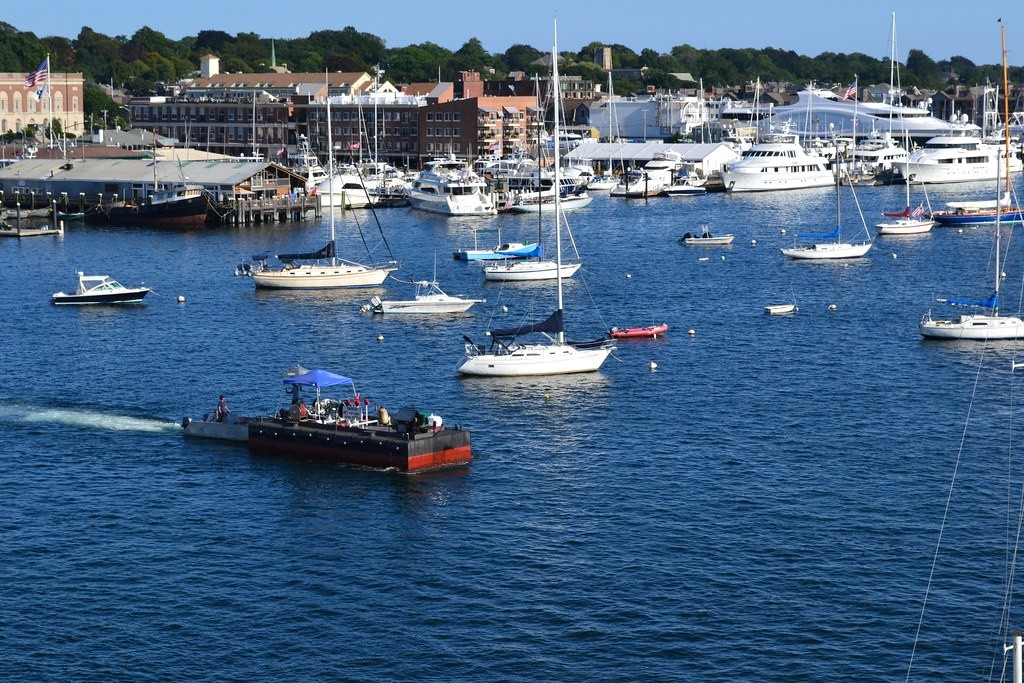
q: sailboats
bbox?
[450,48,620,380]
[922,25,1024,228]
[249,94,404,291]
[481,15,585,281]
[916,150,1024,342]
[777,143,877,260]
[873,131,937,236]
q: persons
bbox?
[218,394,229,421]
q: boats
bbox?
[306,9,1024,218]
[678,223,736,248]
[82,126,210,229]
[51,269,151,307]
[52,209,84,222]
[357,248,488,315]
[608,322,669,339]
[179,362,472,477]
[451,120,543,263]
[762,304,795,316]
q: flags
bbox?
[24,59,47,87]
[912,204,923,218]
[350,143,359,150]
[842,81,857,102]
[32,85,47,102]
[489,141,499,150]
[276,148,284,155]
